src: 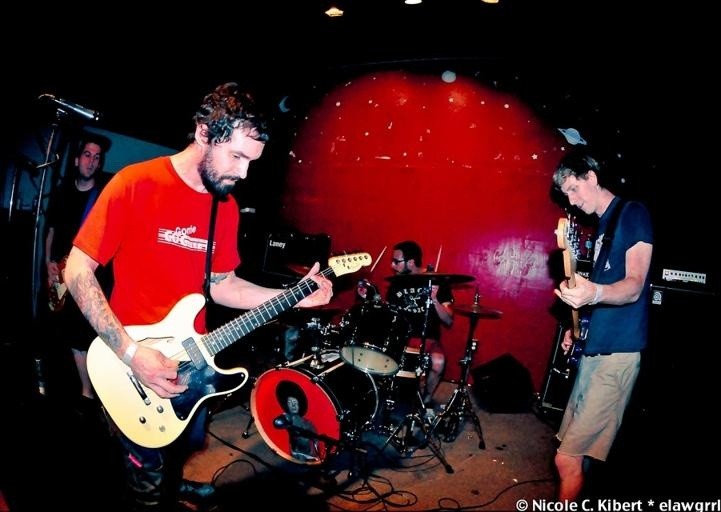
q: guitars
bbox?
[47,253,68,313]
[554,213,592,382]
[86,249,372,449]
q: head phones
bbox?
[196,106,229,137]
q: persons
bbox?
[355,238,455,414]
[31,131,113,400]
[60,80,336,511]
[552,147,655,511]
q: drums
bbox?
[394,347,429,379]
[338,300,413,380]
[249,346,378,465]
[297,318,342,359]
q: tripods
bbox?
[428,319,486,451]
[373,299,454,474]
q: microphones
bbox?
[272,416,288,430]
[99,405,144,469]
[51,95,104,122]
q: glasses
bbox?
[392,258,404,266]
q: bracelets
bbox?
[121,339,140,365]
[588,282,604,305]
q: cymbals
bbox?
[385,272,475,285]
[301,265,361,285]
[447,303,504,319]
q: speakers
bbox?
[470,353,535,413]
[259,231,332,279]
